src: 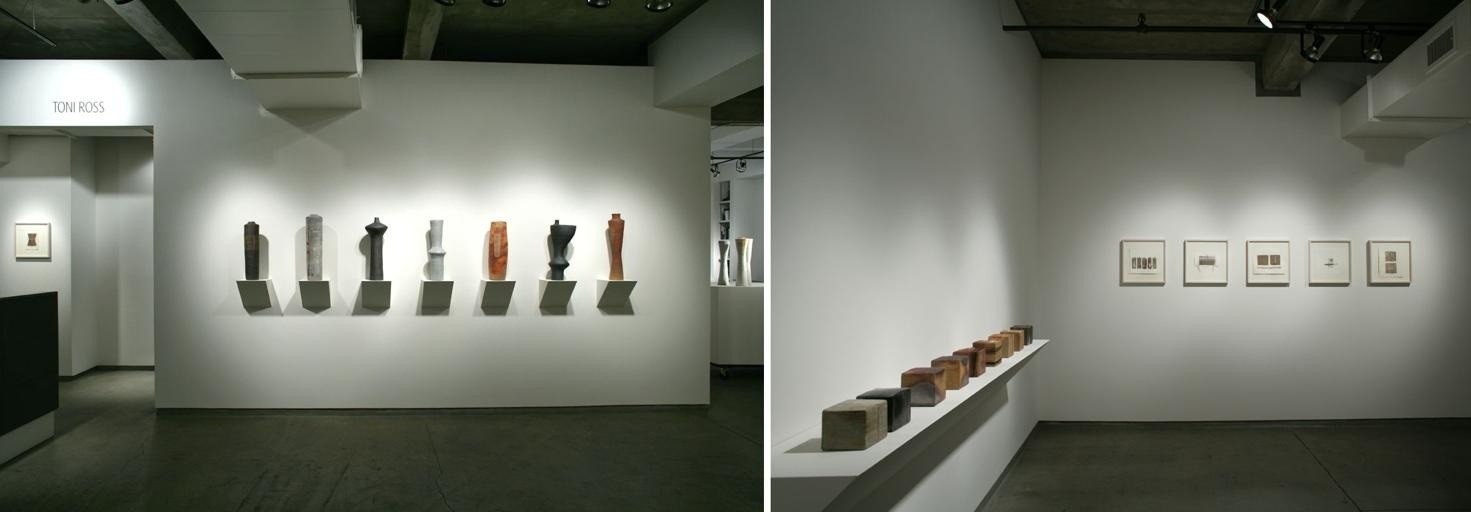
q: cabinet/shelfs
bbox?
[720,179,765,281]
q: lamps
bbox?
[710,138,765,178]
[1256,1,1402,66]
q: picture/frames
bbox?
[12,220,55,260]
[1245,238,1290,285]
[1184,239,1229,284]
[1118,235,1167,287]
[1307,239,1354,287]
[1367,240,1412,285]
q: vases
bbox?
[365,216,389,279]
[547,220,576,280]
[243,221,262,280]
[305,214,325,280]
[607,213,626,280]
[489,221,508,281]
[427,219,447,280]
[736,238,751,286]
[747,238,755,286]
[718,240,733,285]
